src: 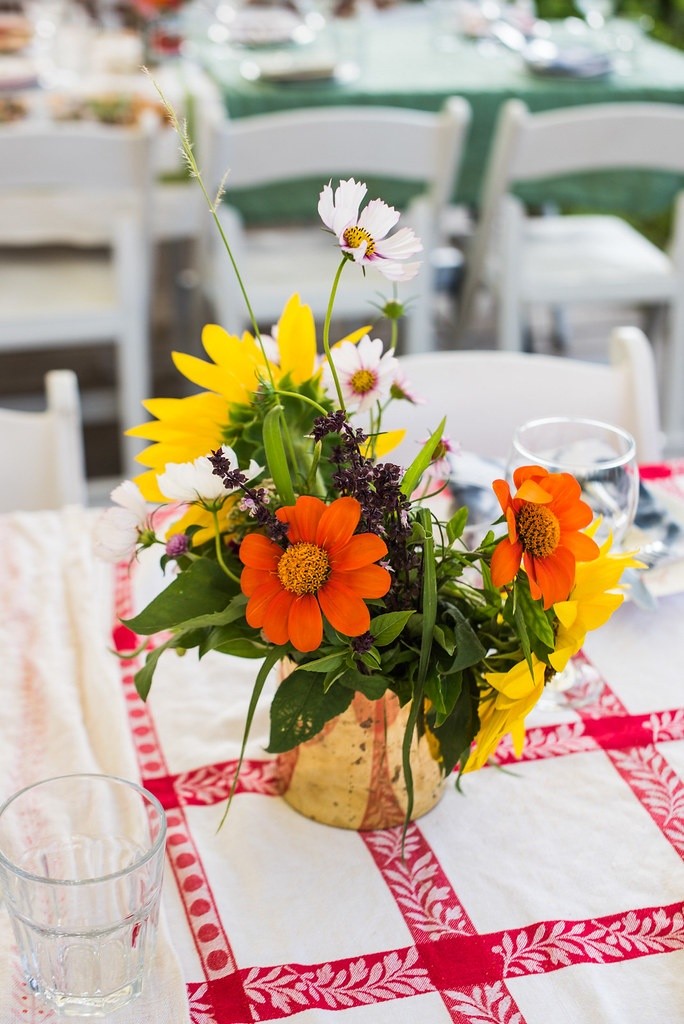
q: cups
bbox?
[0,773,167,1018]
[504,415,640,554]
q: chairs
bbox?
[0,0,684,517]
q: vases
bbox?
[274,651,450,835]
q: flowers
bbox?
[92,51,668,876]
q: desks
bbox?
[203,16,683,248]
[0,452,684,1024]
